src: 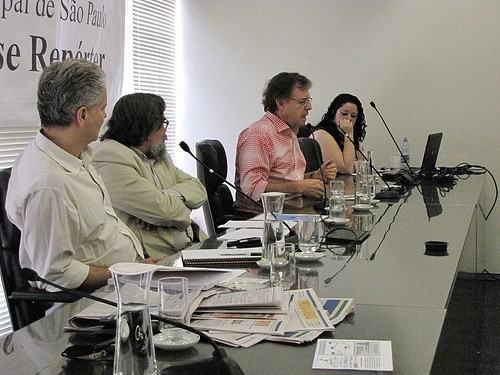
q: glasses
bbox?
[162,119,169,128]
[61,341,114,361]
[288,97,312,107]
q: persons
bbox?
[90,93,207,259]
[5,57,168,317]
[227,72,338,220]
[308,93,368,175]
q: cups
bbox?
[328,179,345,222]
[369,174,377,200]
[157,277,190,340]
[297,217,321,255]
[269,241,296,291]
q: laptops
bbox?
[404,133,443,177]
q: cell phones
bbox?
[227,237,262,248]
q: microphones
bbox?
[326,118,401,200]
[306,122,329,214]
[370,101,417,181]
[20,267,244,375]
[179,141,298,243]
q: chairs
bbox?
[296,124,324,174]
[195,139,234,238]
[0,167,83,331]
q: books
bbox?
[181,246,262,268]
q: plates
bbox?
[290,252,326,262]
[350,203,375,212]
[322,217,350,225]
[256,258,289,271]
[324,205,346,212]
[371,199,381,205]
[223,280,267,292]
[152,331,201,350]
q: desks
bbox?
[0,166,487,375]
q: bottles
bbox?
[261,191,287,264]
[354,160,371,206]
[400,137,410,170]
[106,260,160,375]
